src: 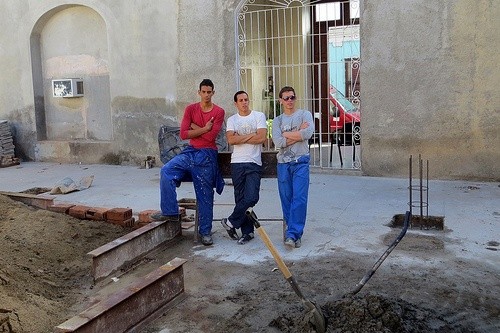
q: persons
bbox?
[150,78,225,245]
[221,91,267,244]
[272,86,315,249]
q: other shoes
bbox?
[201,234,214,245]
[221,218,255,245]
[283,234,302,249]
[149,213,180,222]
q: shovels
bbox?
[246,207,325,333]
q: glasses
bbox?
[281,95,296,101]
